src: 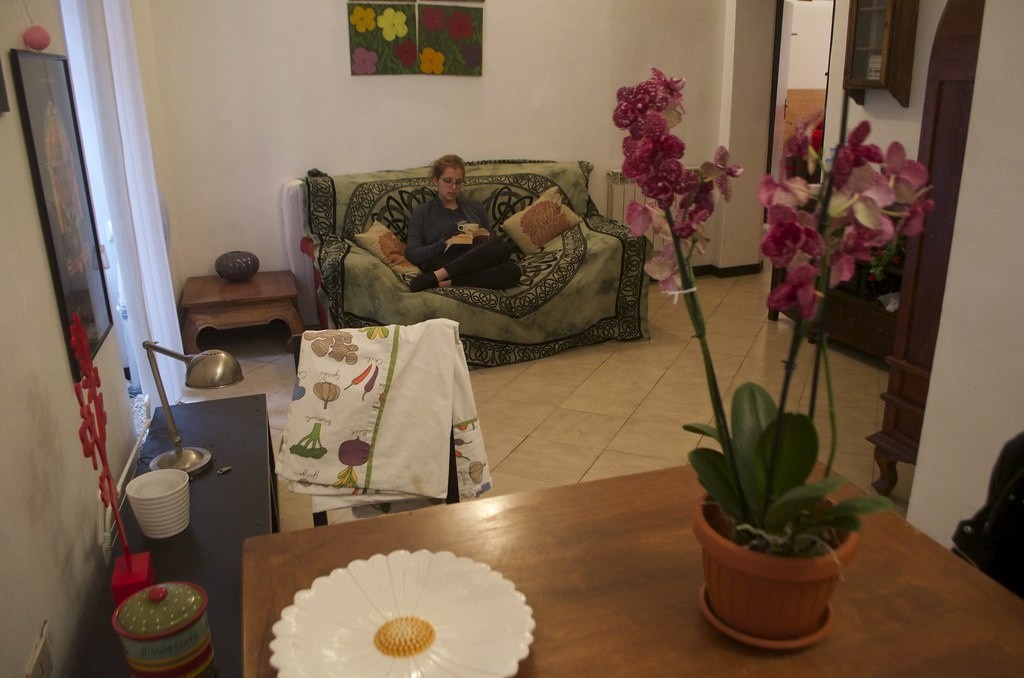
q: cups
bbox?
[125,469,190,539]
[112,581,218,678]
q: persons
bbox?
[404,155,523,293]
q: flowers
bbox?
[612,66,935,586]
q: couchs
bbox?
[281,160,655,371]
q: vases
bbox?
[691,492,861,656]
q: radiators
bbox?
[606,166,700,258]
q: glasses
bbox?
[439,177,464,189]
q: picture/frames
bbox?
[9,47,114,384]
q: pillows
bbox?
[500,185,583,256]
[353,219,422,280]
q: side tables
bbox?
[179,270,305,355]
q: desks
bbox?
[241,460,1024,678]
[62,393,281,678]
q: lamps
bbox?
[142,339,245,479]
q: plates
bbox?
[269,550,537,678]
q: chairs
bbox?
[274,316,494,527]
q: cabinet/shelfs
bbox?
[768,185,906,369]
[843,0,920,108]
